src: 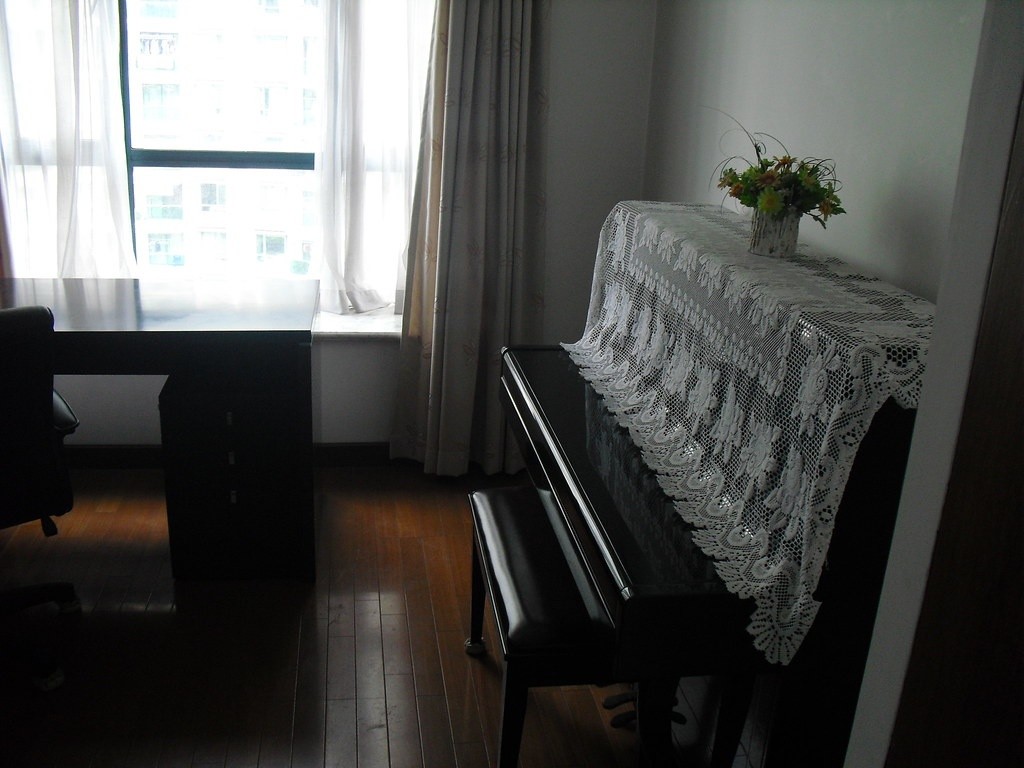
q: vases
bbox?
[747,205,801,260]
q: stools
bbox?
[456,486,674,768]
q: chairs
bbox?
[1,304,81,622]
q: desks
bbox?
[2,274,324,626]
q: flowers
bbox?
[700,102,850,231]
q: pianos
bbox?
[500,200,939,767]
